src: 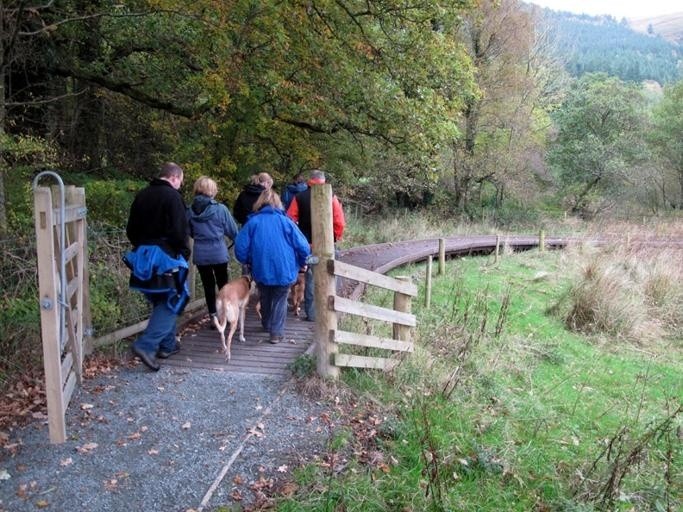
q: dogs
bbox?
[254,264,306,321]
[211,272,255,363]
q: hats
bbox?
[310,169,324,179]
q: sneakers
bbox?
[270,334,285,344]
[132,343,160,371]
[160,341,180,358]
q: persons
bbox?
[126,162,194,371]
[286,169,344,323]
[234,187,312,343]
[187,174,240,332]
[282,175,309,214]
[231,173,274,276]
